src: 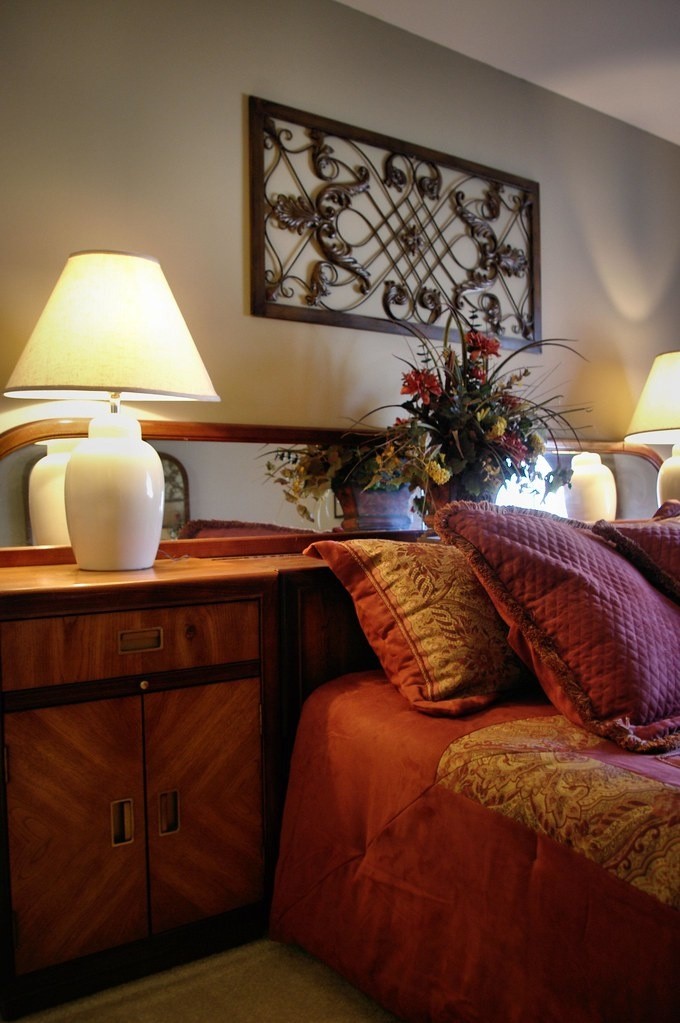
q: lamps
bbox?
[19,437,91,547]
[622,349,680,510]
[564,454,617,525]
[6,252,220,572]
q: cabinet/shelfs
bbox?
[0,577,274,978]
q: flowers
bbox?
[338,323,582,494]
[264,431,417,522]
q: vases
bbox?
[337,482,413,519]
[417,478,496,522]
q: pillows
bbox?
[301,535,511,712]
[435,507,679,751]
[592,512,680,599]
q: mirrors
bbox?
[2,418,661,563]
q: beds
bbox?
[279,501,680,1022]
